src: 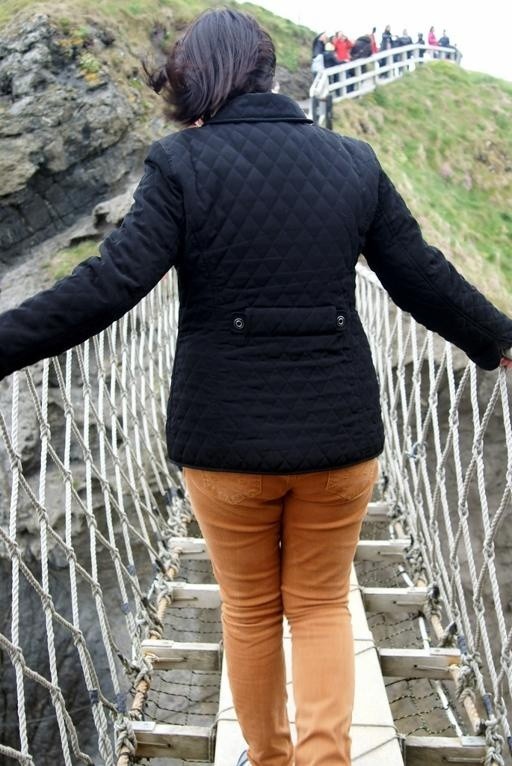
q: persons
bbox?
[309,19,454,95]
[0,8,512,766]
